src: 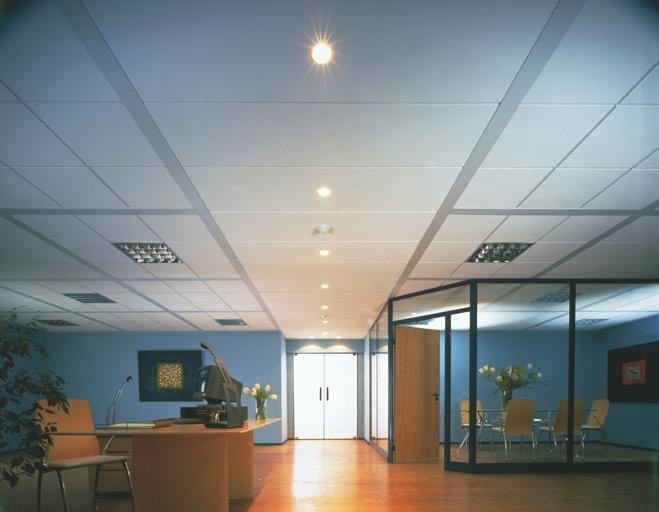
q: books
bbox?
[107,422,156,429]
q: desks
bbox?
[52,418,281,511]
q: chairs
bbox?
[456,400,609,462]
[35,399,134,512]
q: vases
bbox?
[255,397,267,419]
[500,388,512,410]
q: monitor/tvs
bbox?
[193,365,243,407]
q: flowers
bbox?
[243,383,278,400]
[479,362,542,395]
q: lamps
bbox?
[107,376,132,424]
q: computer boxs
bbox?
[181,406,247,421]
